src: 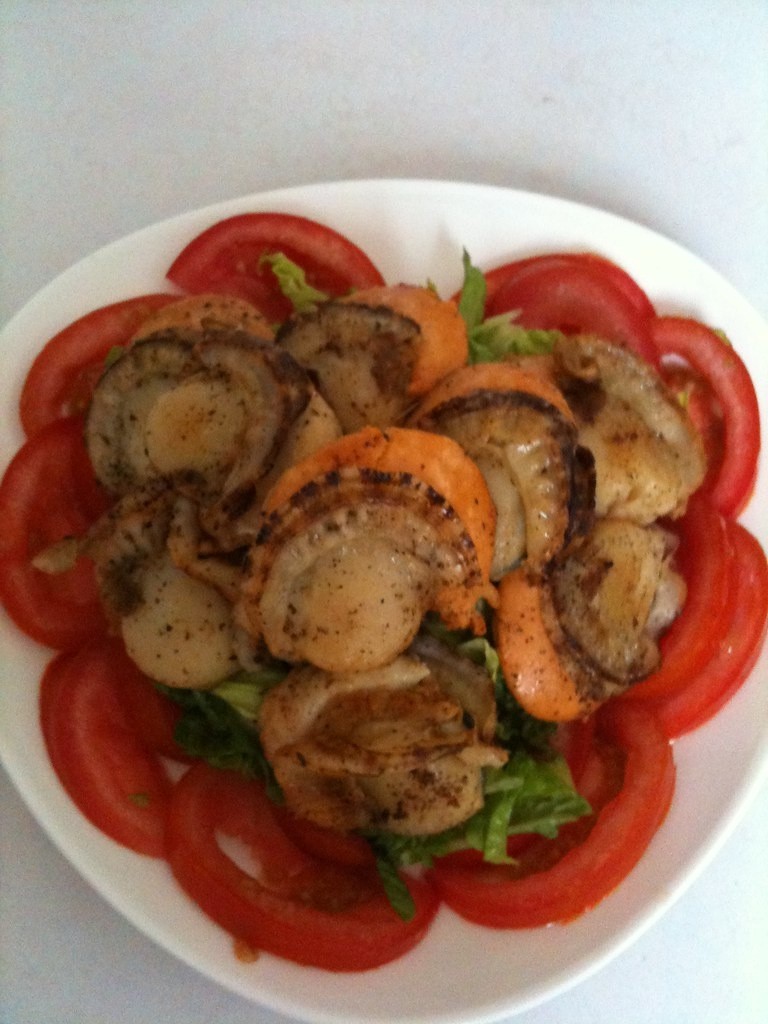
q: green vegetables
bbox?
[149,246,600,923]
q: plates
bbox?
[0,178,768,1024]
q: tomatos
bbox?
[1,212,768,973]
